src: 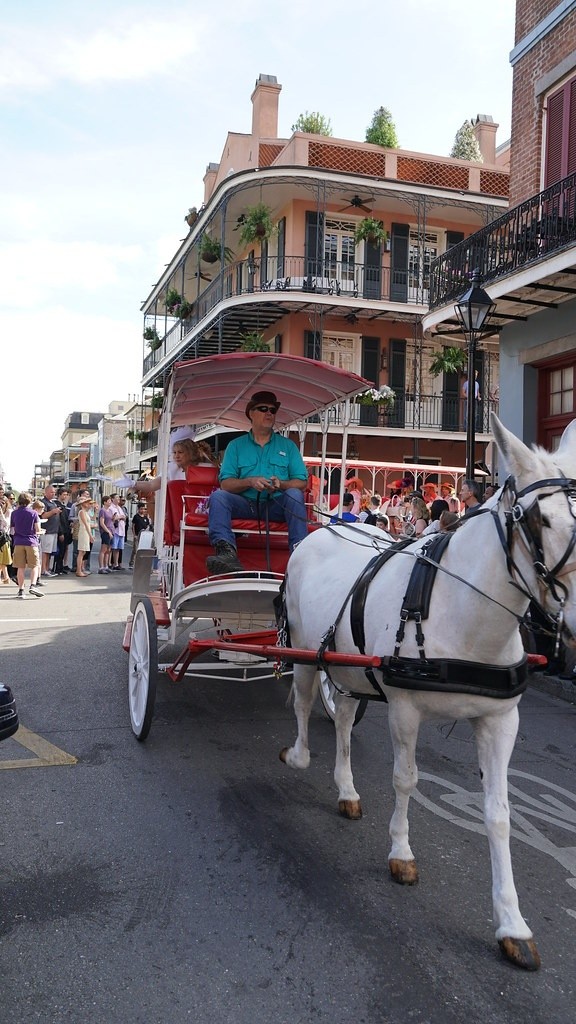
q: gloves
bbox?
[111,473,137,488]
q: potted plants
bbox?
[143,325,161,350]
[150,392,164,408]
[124,429,149,440]
[353,217,389,250]
[196,232,237,266]
[162,287,186,308]
[238,330,271,352]
[427,345,468,376]
[237,200,282,250]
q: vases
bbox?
[181,308,189,318]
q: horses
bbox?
[277,409,576,971]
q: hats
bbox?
[441,482,452,492]
[343,493,354,502]
[401,478,412,488]
[387,481,397,488]
[420,483,435,492]
[76,496,91,506]
[245,391,280,418]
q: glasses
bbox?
[251,405,278,414]
[7,497,15,501]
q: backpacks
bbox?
[364,509,383,527]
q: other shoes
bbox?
[106,568,114,573]
[54,570,67,576]
[113,566,125,572]
[18,589,23,598]
[48,570,59,576]
[129,562,134,569]
[41,570,55,578]
[0,577,10,585]
[71,566,77,572]
[98,568,108,574]
[29,586,44,597]
[76,571,88,577]
[85,566,90,571]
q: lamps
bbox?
[381,347,388,369]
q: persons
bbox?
[0,487,98,584]
[98,496,116,574]
[376,517,388,530]
[11,494,44,597]
[113,425,219,492]
[462,370,482,432]
[329,476,362,522]
[206,392,309,576]
[75,497,98,576]
[109,493,126,570]
[118,496,129,567]
[128,504,152,569]
[386,477,501,537]
[359,495,389,531]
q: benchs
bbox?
[164,465,323,549]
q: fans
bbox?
[338,195,376,213]
[185,264,213,282]
[331,313,376,328]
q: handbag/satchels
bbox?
[72,521,80,540]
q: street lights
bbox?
[453,266,499,514]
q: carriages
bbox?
[122,351,576,971]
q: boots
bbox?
[206,540,245,574]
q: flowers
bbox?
[172,298,193,318]
[362,385,396,413]
